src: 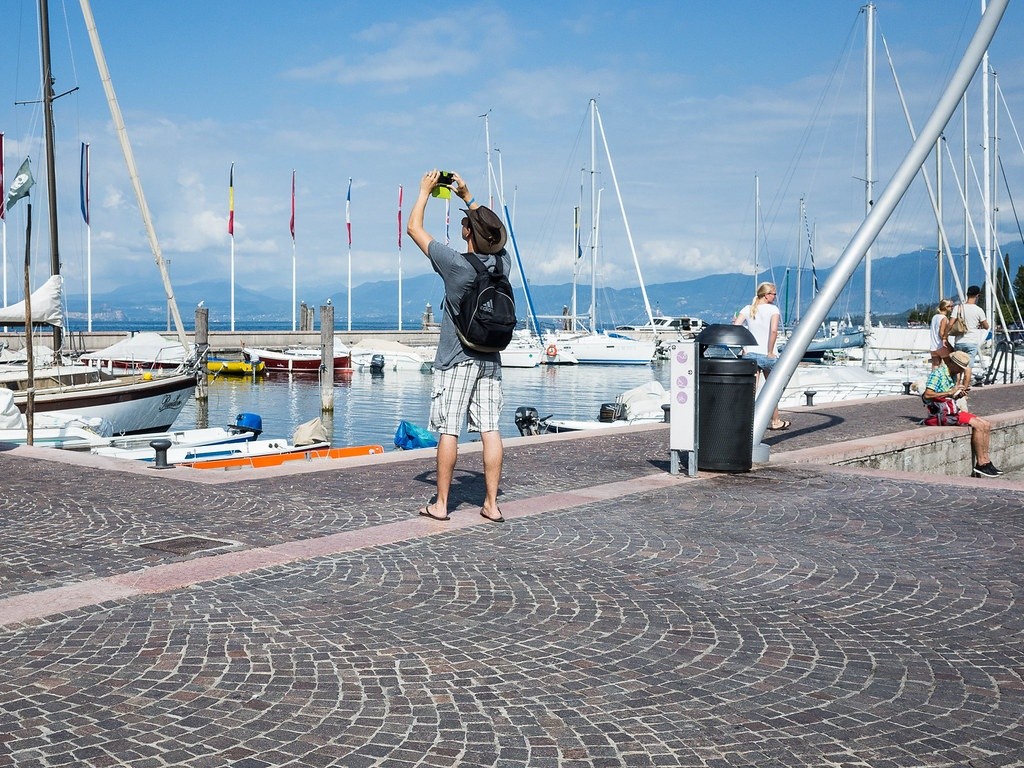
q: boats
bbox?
[169,417,437,471]
[244,341,354,371]
[74,330,198,371]
[206,344,265,374]
[286,334,437,371]
[0,410,266,456]
[101,415,334,464]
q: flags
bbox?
[5,159,34,212]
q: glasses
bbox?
[767,293,776,295]
[946,305,953,308]
[460,217,468,225]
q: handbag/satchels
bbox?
[950,304,967,335]
[922,397,958,425]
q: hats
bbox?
[968,286,981,296]
[459,205,507,254]
[949,351,969,369]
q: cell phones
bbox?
[963,389,971,392]
[437,171,454,185]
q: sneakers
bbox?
[973,463,999,478]
[989,461,1003,474]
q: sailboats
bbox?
[475,1,1024,438]
[0,0,211,437]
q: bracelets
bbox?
[467,198,475,206]
[943,337,947,338]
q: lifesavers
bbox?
[547,346,557,356]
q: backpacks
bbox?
[440,252,516,353]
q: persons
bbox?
[929,299,954,372]
[923,351,1004,478]
[943,286,989,392]
[406,169,511,526]
[734,281,791,430]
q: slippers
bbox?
[480,506,505,522]
[770,421,791,430]
[420,506,449,520]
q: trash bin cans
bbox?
[694,323,758,474]
